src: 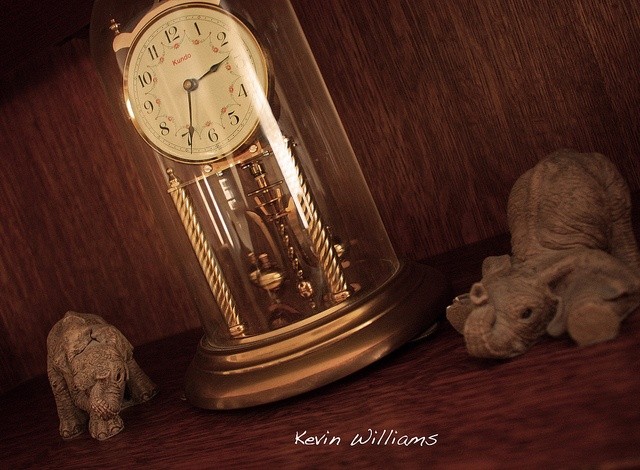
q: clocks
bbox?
[86,0,463,412]
